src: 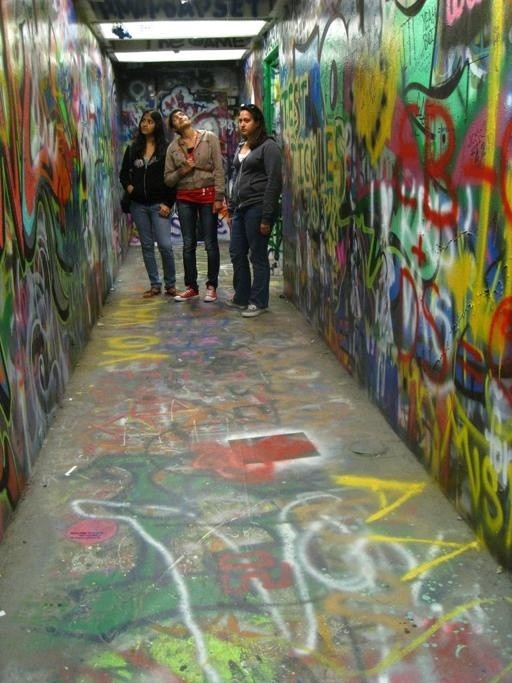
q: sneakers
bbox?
[174,284,199,302]
[241,304,269,317]
[225,299,247,309]
[204,284,217,302]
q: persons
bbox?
[163,110,226,302]
[225,104,283,317]
[119,110,182,296]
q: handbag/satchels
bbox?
[120,191,132,212]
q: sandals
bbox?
[143,288,161,298]
[166,287,184,295]
